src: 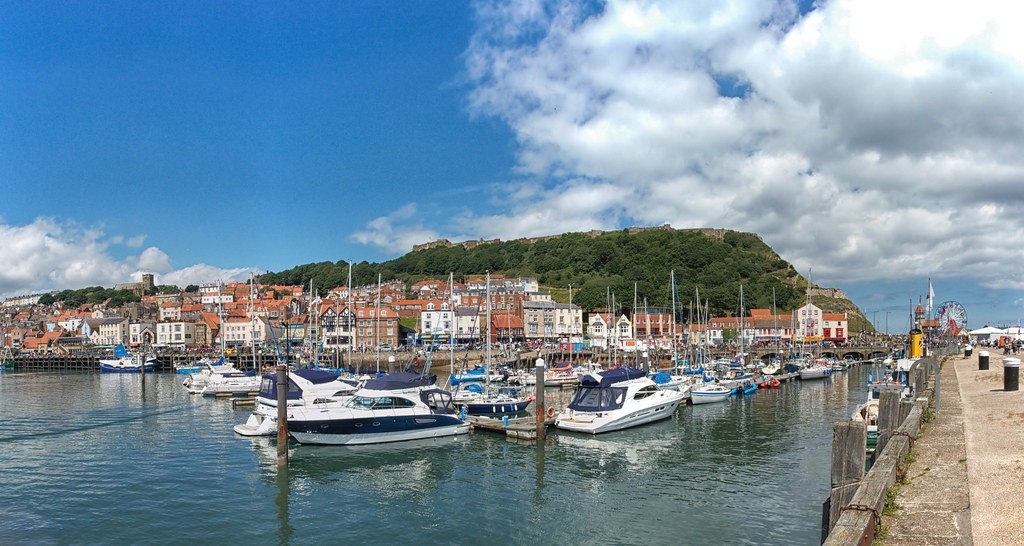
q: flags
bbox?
[929,278,935,312]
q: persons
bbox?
[986,337,991,348]
[1012,338,1022,355]
[994,338,998,346]
[971,339,974,347]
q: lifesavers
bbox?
[546,405,556,418]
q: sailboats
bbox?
[97,261,922,447]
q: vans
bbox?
[437,344,450,351]
[449,344,465,351]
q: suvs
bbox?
[376,345,392,353]
